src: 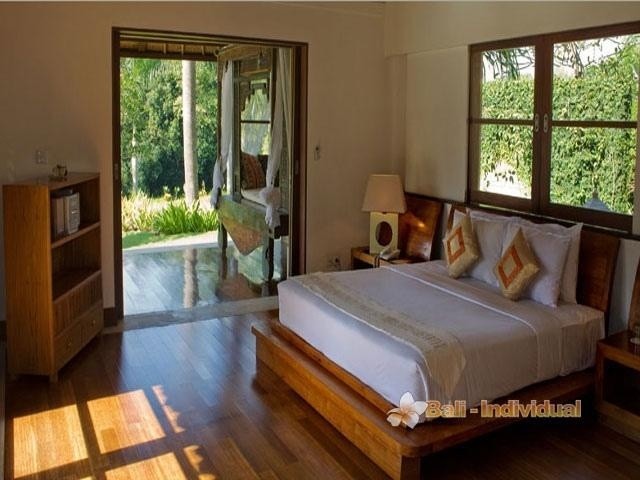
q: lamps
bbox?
[361,175,410,257]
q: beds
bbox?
[207,43,292,282]
[249,196,622,480]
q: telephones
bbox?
[378,245,401,261]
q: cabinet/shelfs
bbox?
[1,169,106,387]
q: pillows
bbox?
[464,205,518,224]
[257,153,279,187]
[239,150,267,191]
[516,215,586,306]
[492,223,542,301]
[501,218,574,310]
[441,210,483,282]
[450,207,511,287]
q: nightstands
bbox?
[350,191,445,270]
[594,259,640,445]
